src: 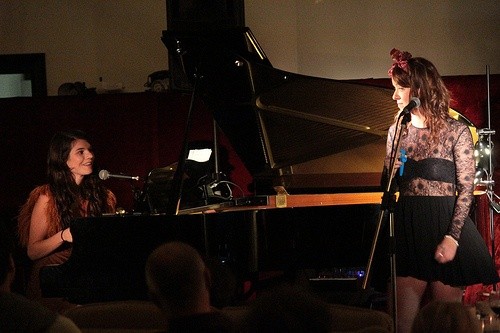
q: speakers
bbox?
[166,0,245,82]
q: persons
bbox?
[373,49,500,333]
[0,238,85,333]
[415,299,499,333]
[245,283,340,333]
[17,128,120,308]
[144,244,239,333]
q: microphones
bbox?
[98,170,140,181]
[400,97,421,116]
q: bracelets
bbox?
[445,236,459,247]
[60,228,65,242]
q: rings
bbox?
[439,253,444,257]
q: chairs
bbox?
[69,300,393,333]
